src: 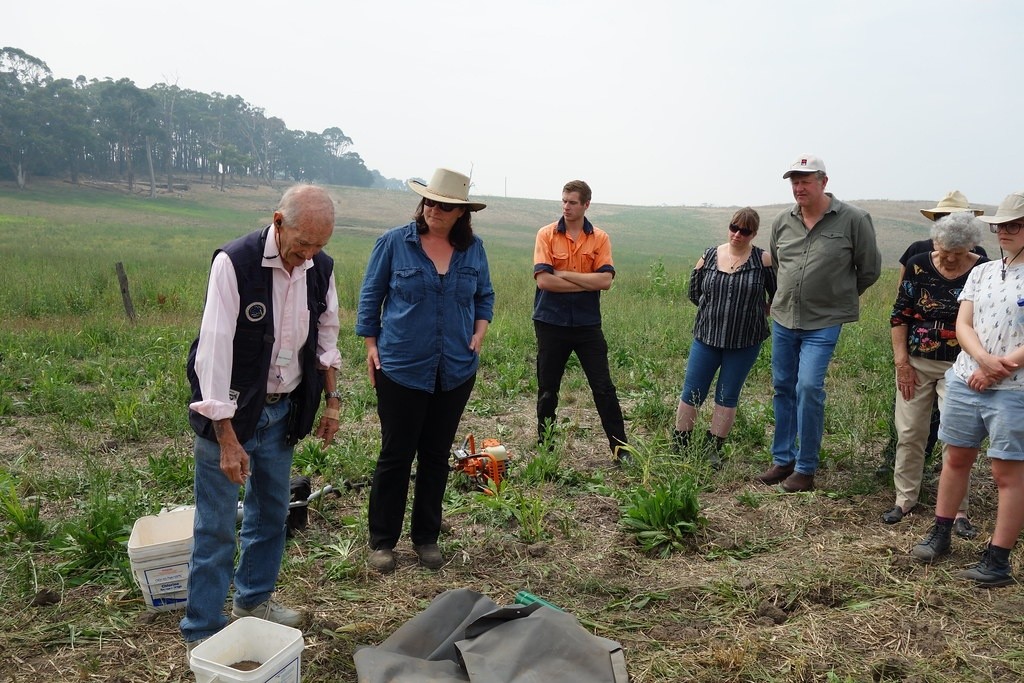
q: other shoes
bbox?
[882,504,916,523]
[876,453,896,476]
[701,447,723,470]
[611,452,634,468]
[953,518,976,539]
[669,447,688,460]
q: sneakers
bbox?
[958,544,1014,586]
[231,598,302,626]
[911,519,955,562]
[186,636,211,664]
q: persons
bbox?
[877,191,987,476]
[883,213,991,538]
[673,208,775,471]
[180,186,343,667]
[912,192,1024,586]
[754,156,881,492]
[356,168,496,571]
[533,180,632,463]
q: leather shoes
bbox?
[783,471,814,492]
[367,546,394,572]
[411,541,444,567]
[754,461,796,484]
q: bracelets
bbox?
[325,392,341,401]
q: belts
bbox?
[264,393,292,405]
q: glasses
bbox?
[990,222,1024,235]
[422,197,465,212]
[729,224,755,237]
[933,213,946,221]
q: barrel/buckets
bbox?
[189,616,305,683]
[128,509,195,614]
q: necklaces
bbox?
[727,244,750,269]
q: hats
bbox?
[782,154,827,179]
[408,168,486,212]
[920,189,984,223]
[978,191,1024,225]
